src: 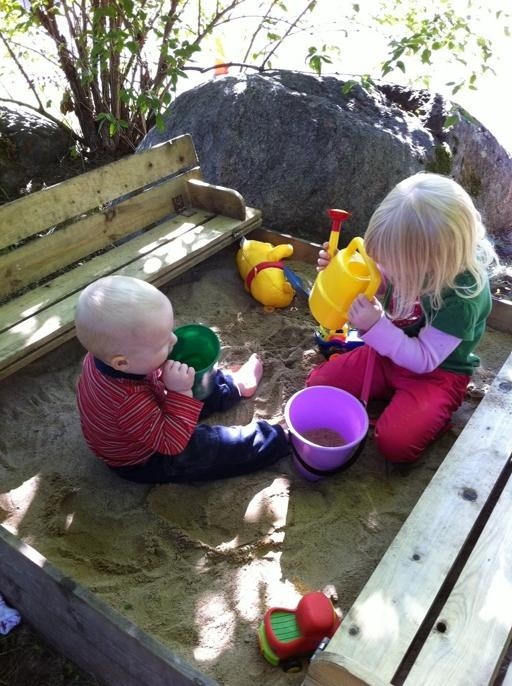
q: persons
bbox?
[309,171,503,464]
[72,273,295,485]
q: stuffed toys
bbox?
[235,235,297,313]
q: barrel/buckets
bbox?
[168,322,221,402]
[285,386,370,476]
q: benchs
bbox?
[0,133,262,380]
[304,349,511,685]
